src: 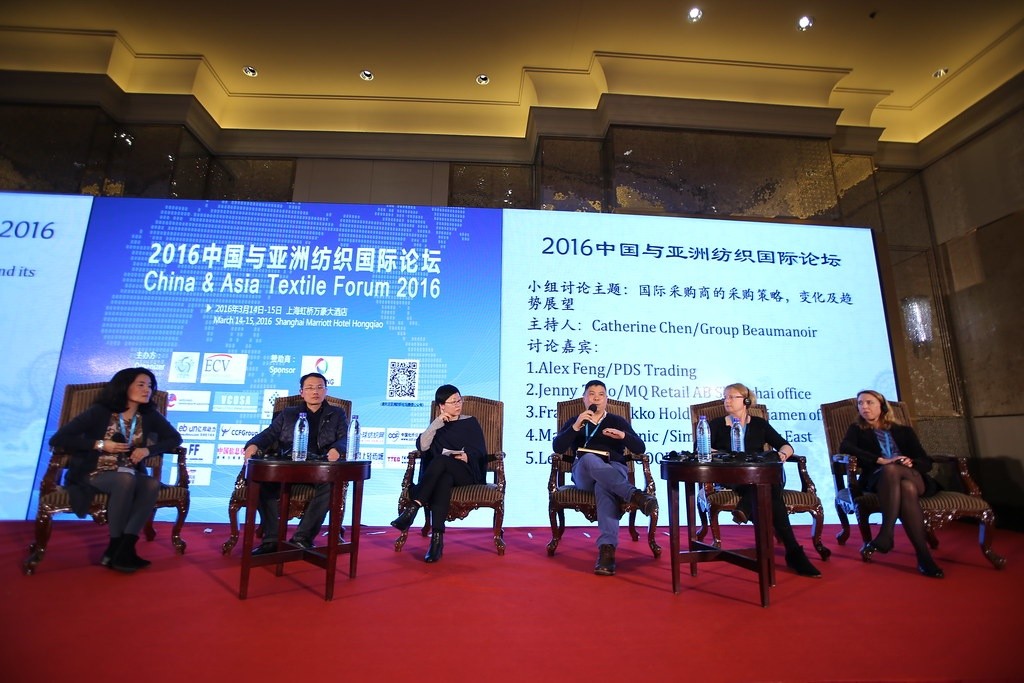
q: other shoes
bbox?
[918,564,944,577]
[867,538,893,554]
[786,545,822,577]
[731,499,752,524]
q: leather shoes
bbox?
[631,489,657,517]
[289,539,316,549]
[594,544,617,574]
[250,542,277,554]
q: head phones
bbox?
[662,451,690,459]
[738,451,758,463]
[743,389,751,405]
[857,394,889,413]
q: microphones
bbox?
[111,433,131,457]
[582,404,597,426]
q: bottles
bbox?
[697,416,712,462]
[731,418,744,452]
[292,413,309,462]
[346,415,360,461]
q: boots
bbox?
[101,533,151,572]
[391,504,419,531]
[424,533,443,562]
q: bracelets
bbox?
[97,440,104,450]
[781,451,787,458]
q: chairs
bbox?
[690,401,833,562]
[21,384,190,576]
[393,396,507,563]
[820,397,1008,570]
[220,394,351,552]
[548,399,661,559]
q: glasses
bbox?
[303,385,327,393]
[723,396,744,401]
[444,399,463,405]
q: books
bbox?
[577,447,610,463]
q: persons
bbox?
[838,390,945,579]
[48,368,182,571]
[709,382,821,578]
[242,373,347,553]
[390,383,487,563]
[553,380,657,575]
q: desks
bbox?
[662,454,783,607]
[237,460,372,601]
